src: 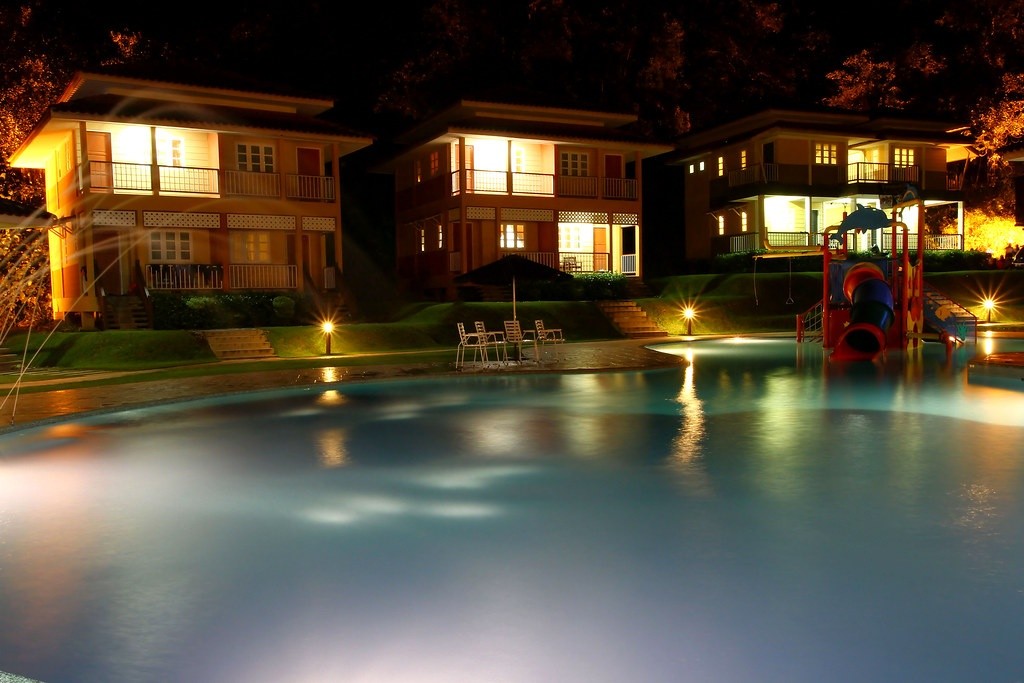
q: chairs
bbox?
[456,322,490,370]
[563,256,583,275]
[502,320,540,365]
[475,321,509,369]
[535,319,567,364]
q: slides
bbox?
[828,263,895,360]
